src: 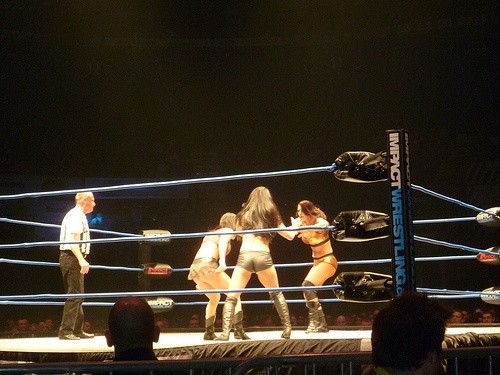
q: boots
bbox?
[314,302,329,332]
[203,314,218,341]
[301,280,320,334]
[214,296,237,341]
[269,290,292,339]
[232,310,251,340]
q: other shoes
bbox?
[74,330,95,338]
[59,333,81,340]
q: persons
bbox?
[290,200,338,334]
[59,192,96,339]
[361,291,447,375]
[212,186,302,341]
[0,316,91,338]
[189,303,497,330]
[104,296,160,360]
[187,212,251,340]
